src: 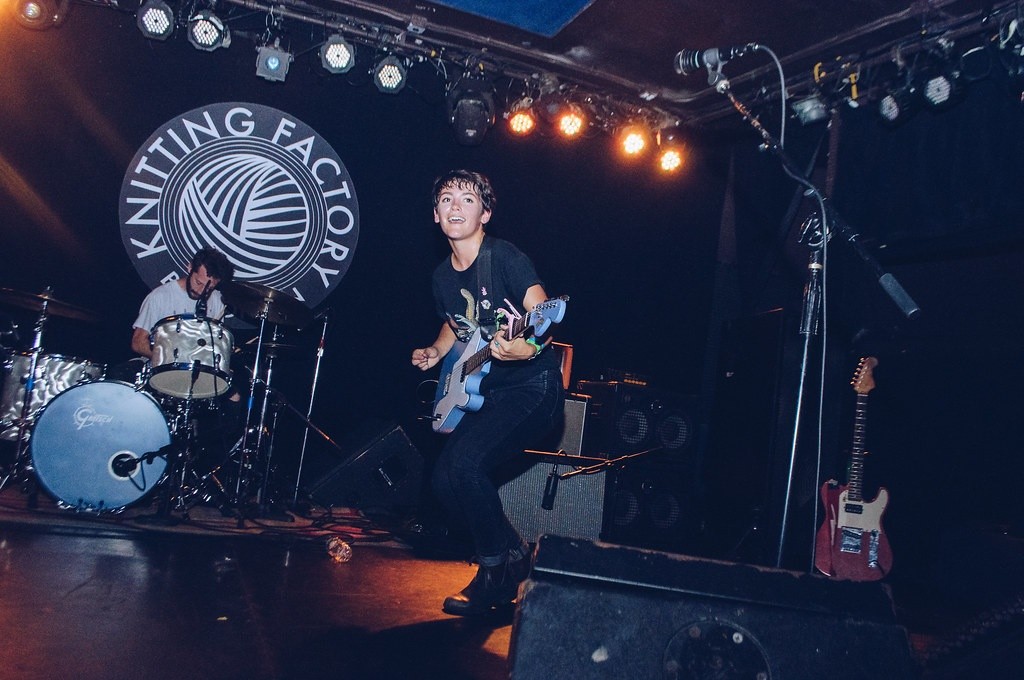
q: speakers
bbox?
[303,424,431,513]
[576,380,703,546]
[508,532,920,679]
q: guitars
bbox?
[430,293,570,434]
[811,356,894,581]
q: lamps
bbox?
[739,15,1024,120]
[137,0,175,41]
[10,0,74,29]
[254,17,293,82]
[186,10,231,50]
[443,72,498,144]
[502,91,541,140]
[318,30,355,75]
[368,53,408,95]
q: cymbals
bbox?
[240,342,299,353]
[0,287,104,322]
[222,280,314,328]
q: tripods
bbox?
[0,280,331,530]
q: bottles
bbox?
[326,536,352,563]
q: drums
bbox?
[0,349,109,445]
[148,313,236,400]
[31,378,174,512]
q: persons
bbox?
[132,248,241,518]
[412,168,564,615]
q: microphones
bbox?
[196,280,212,310]
[113,458,127,472]
[296,304,333,337]
[671,42,758,77]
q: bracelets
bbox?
[525,337,545,360]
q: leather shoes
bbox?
[510,550,531,582]
[443,560,518,615]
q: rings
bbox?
[495,342,500,348]
